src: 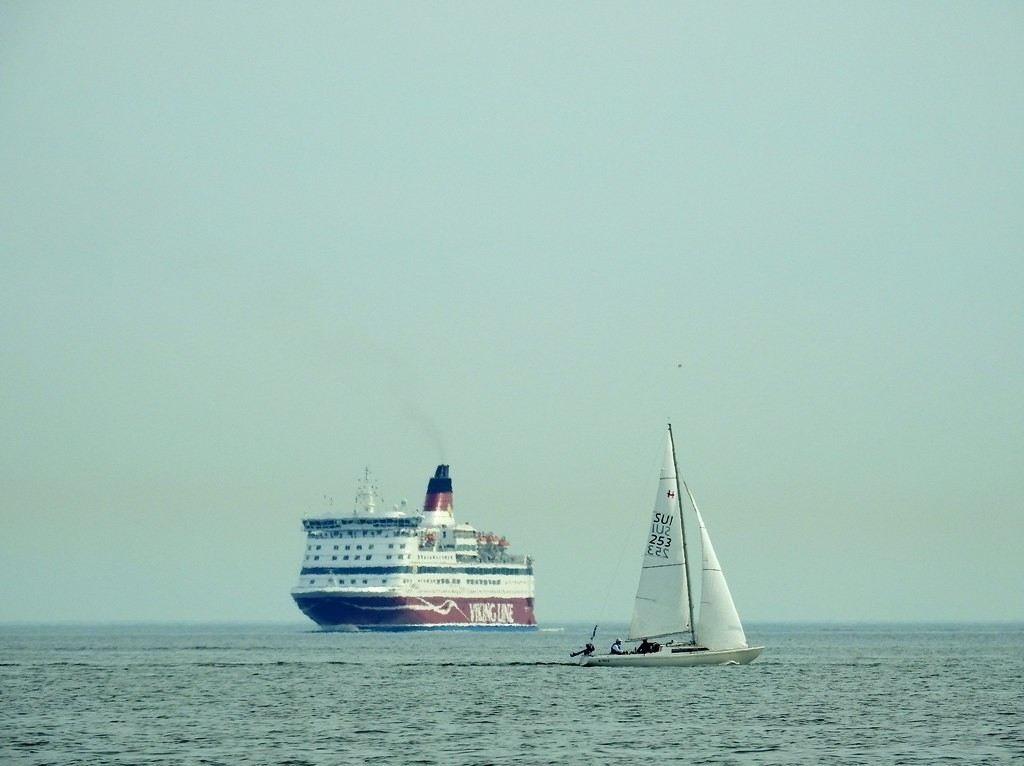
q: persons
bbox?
[611,638,625,654]
[637,639,654,652]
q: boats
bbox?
[290,462,540,631]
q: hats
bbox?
[616,638,622,642]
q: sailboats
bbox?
[569,421,766,667]
